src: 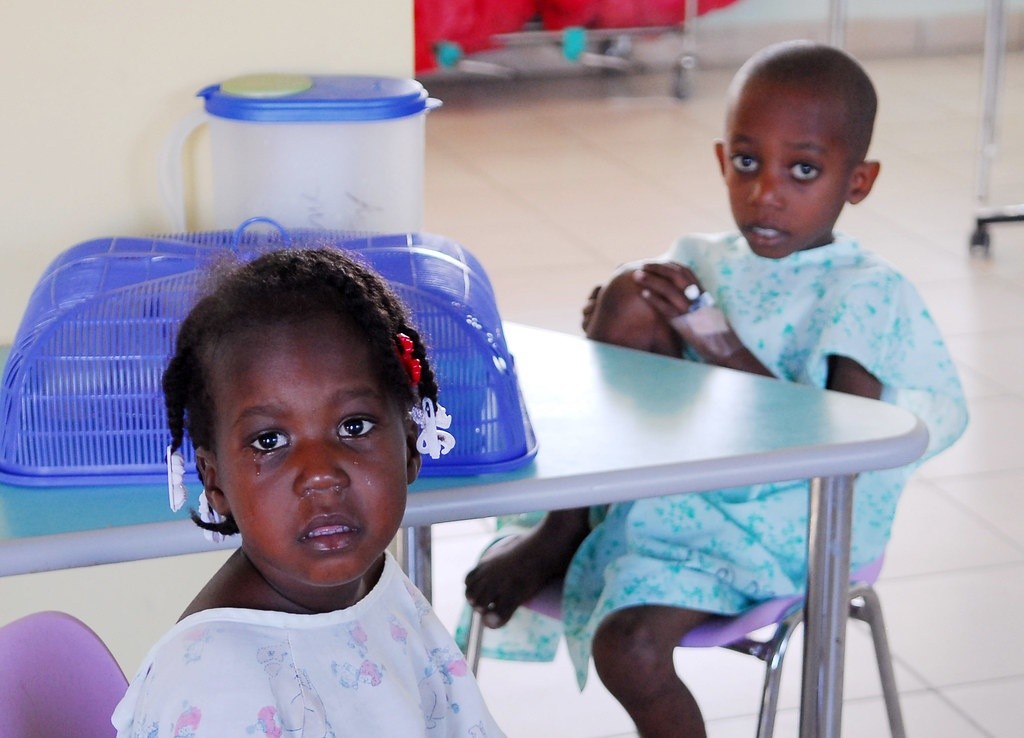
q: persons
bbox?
[464,40,922,738]
[110,246,506,738]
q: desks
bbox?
[0,320,930,738]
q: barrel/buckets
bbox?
[196,73,443,232]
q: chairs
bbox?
[0,611,130,738]
[465,534,908,738]
